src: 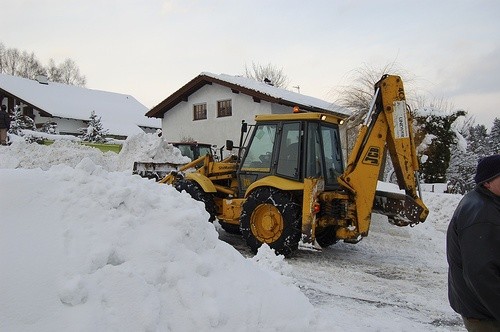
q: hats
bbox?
[474,154,500,185]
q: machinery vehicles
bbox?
[158,71,429,258]
[134,138,218,182]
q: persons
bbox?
[0,105,10,146]
[444,155,500,332]
[287,135,304,160]
